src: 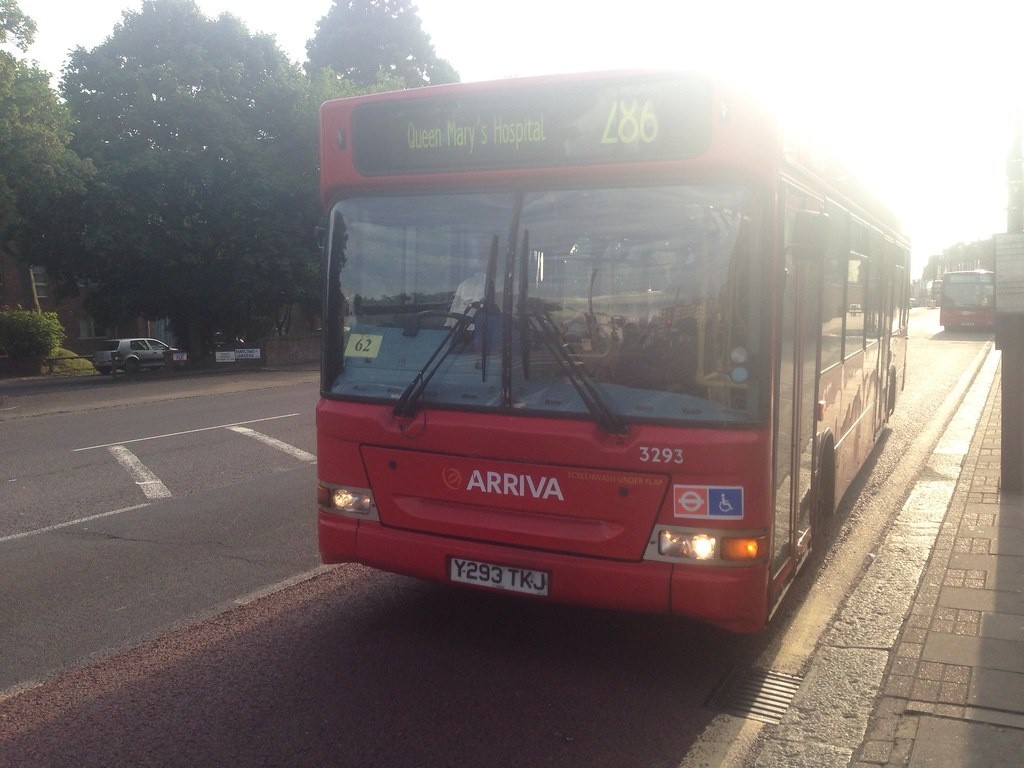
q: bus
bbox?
[939,271,995,333]
[316,72,918,624]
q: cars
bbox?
[94,338,180,374]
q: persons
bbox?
[442,199,535,359]
